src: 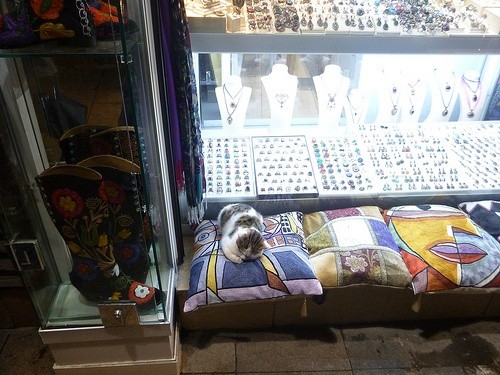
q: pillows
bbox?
[457,198,500,244]
[182,211,322,314]
[383,204,500,297]
[301,204,414,289]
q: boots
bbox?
[78,152,150,278]
[63,124,110,161]
[90,126,159,246]
[35,160,165,311]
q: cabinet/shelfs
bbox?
[0,0,182,375]
[186,30,500,215]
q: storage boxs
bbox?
[225,12,241,32]
[186,11,226,33]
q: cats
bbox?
[216,204,264,264]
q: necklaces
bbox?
[407,78,420,96]
[327,77,344,110]
[438,72,455,117]
[346,95,363,125]
[406,94,415,116]
[222,83,244,125]
[274,94,289,109]
[381,69,405,117]
[462,76,482,118]
[224,87,242,108]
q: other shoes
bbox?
[2,0,139,48]
